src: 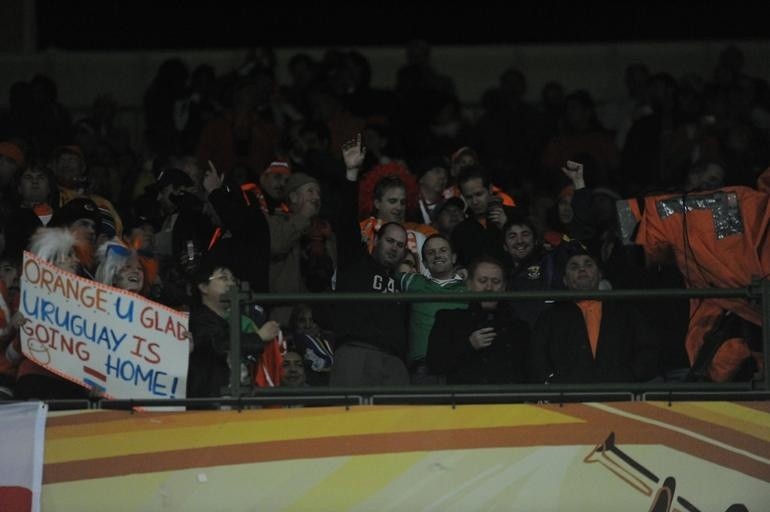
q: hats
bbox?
[261,153,291,173]
[145,167,194,191]
[46,198,100,226]
[559,183,575,198]
[417,155,445,184]
[590,182,622,205]
[561,238,598,268]
[1,141,23,165]
[96,203,116,235]
[432,196,466,219]
[284,171,318,194]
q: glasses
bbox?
[104,244,155,265]
[209,274,239,285]
